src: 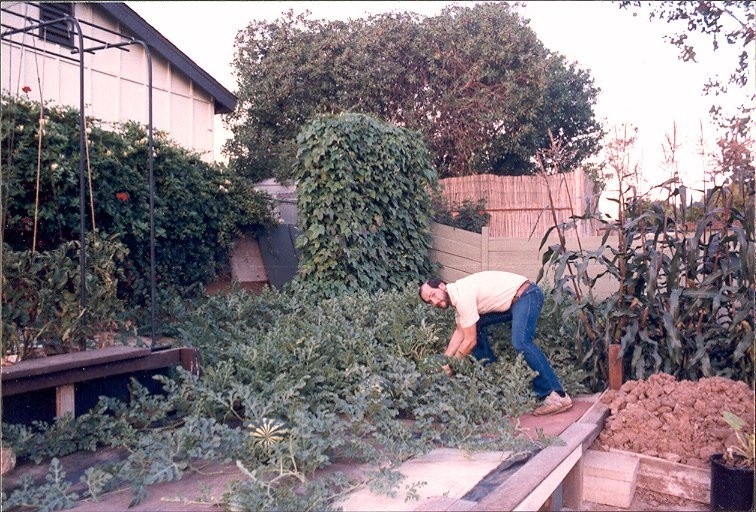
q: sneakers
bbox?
[534,391,573,415]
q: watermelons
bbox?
[246,412,297,464]
[417,354,454,376]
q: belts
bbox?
[512,281,532,303]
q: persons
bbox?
[419,270,573,418]
